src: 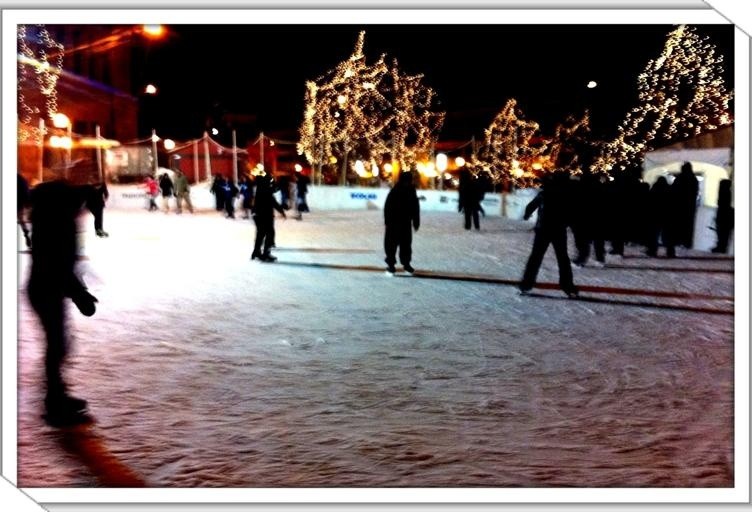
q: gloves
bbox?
[75,289,98,317]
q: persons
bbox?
[210,170,310,262]
[17,172,108,246]
[458,170,486,231]
[174,170,194,214]
[137,176,160,211]
[159,172,174,212]
[516,159,731,295]
[383,170,420,272]
[25,177,98,421]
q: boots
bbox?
[386,263,415,272]
[44,383,88,416]
[251,249,277,262]
